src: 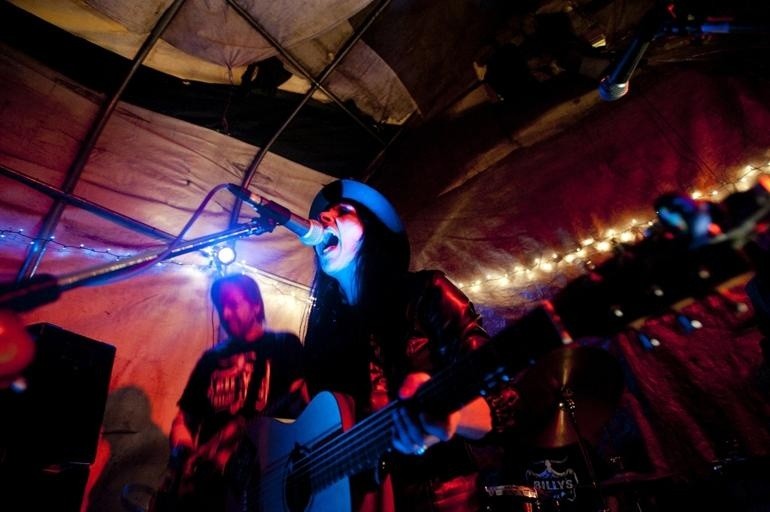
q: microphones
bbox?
[226,183,324,246]
[598,7,673,101]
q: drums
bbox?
[483,485,537,511]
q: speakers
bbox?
[0,322,116,512]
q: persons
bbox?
[301,178,490,512]
[169,272,302,510]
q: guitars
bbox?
[233,300,571,512]
[178,416,249,494]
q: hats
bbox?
[308,179,410,279]
